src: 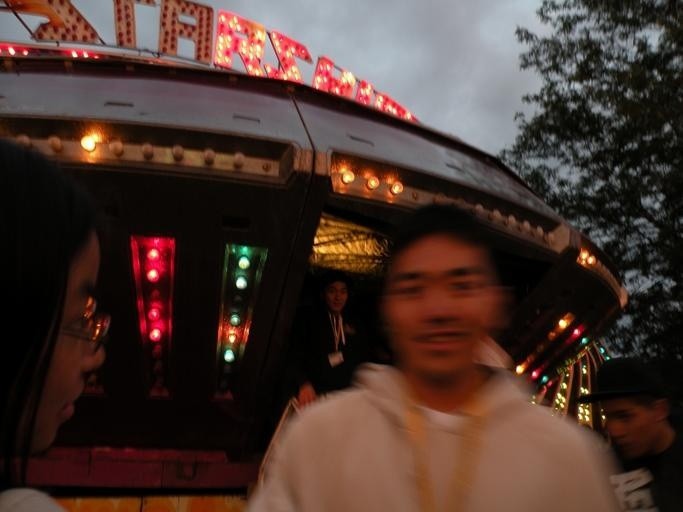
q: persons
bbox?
[287,273,367,408]
[240,200,627,511]
[590,357,681,512]
[0,143,113,510]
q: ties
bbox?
[331,312,344,351]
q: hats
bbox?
[578,355,668,405]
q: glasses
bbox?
[61,310,111,355]
